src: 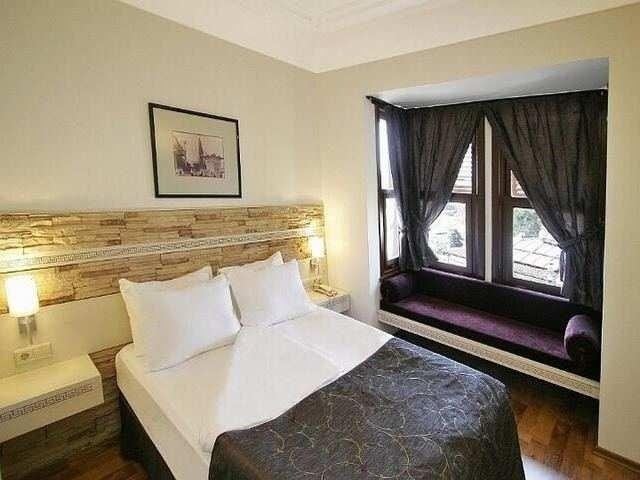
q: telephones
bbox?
[314,285,338,296]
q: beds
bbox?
[115,308,511,480]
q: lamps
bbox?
[5,274,52,366]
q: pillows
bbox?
[119,251,319,374]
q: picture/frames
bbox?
[146,102,241,199]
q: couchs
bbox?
[377,265,601,400]
